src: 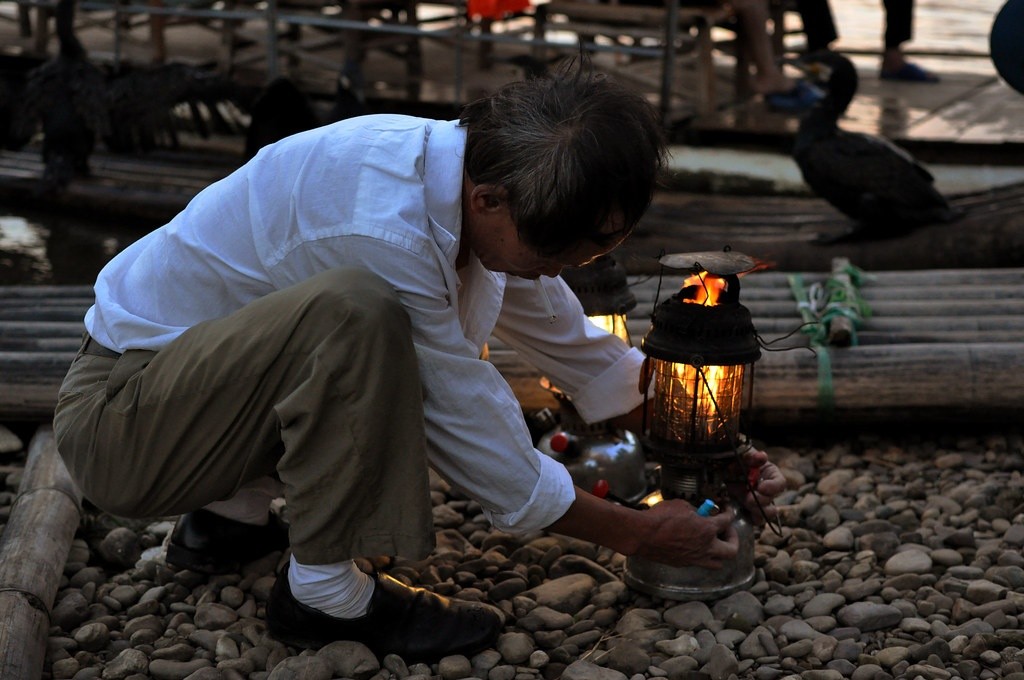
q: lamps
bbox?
[540,254,765,597]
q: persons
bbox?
[730,0,940,111]
[51,56,785,663]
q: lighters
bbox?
[697,499,719,517]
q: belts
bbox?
[81,331,122,361]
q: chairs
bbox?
[0,0,784,135]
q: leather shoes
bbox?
[164,507,288,572]
[262,560,505,665]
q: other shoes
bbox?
[801,45,837,63]
[880,62,939,82]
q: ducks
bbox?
[782,47,966,246]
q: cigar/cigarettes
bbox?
[533,277,555,322]
